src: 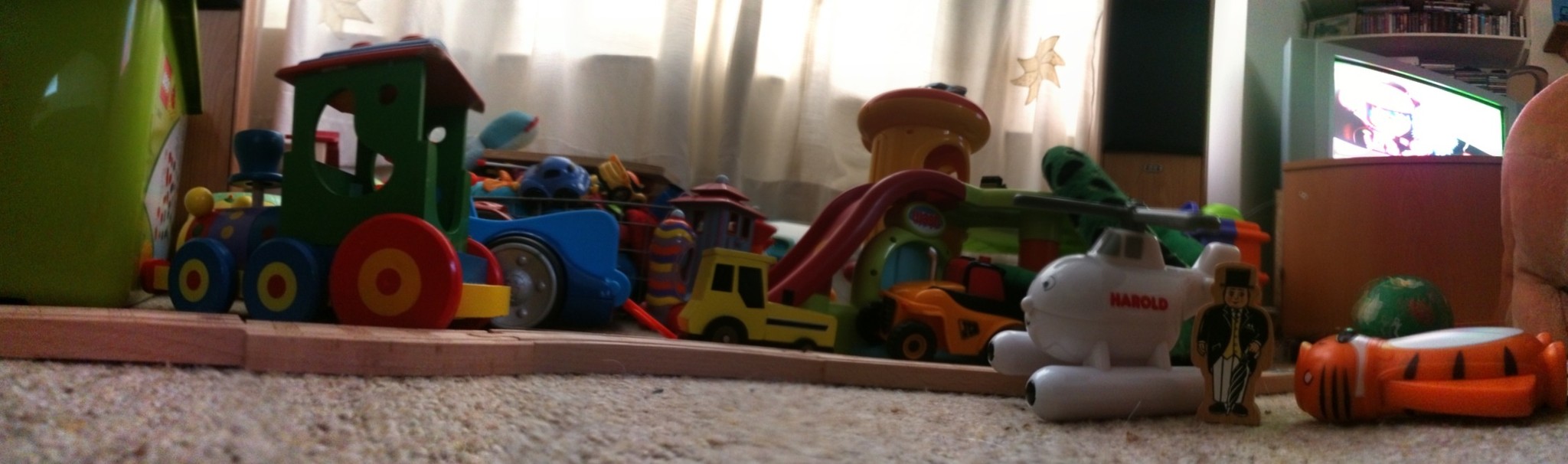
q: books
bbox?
[1353,0,1529,41]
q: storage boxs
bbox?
[0,1,204,309]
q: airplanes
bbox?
[985,231,1242,416]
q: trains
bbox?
[167,32,509,328]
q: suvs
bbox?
[869,257,1025,364]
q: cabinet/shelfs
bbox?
[1297,0,1535,98]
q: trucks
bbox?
[677,250,839,351]
